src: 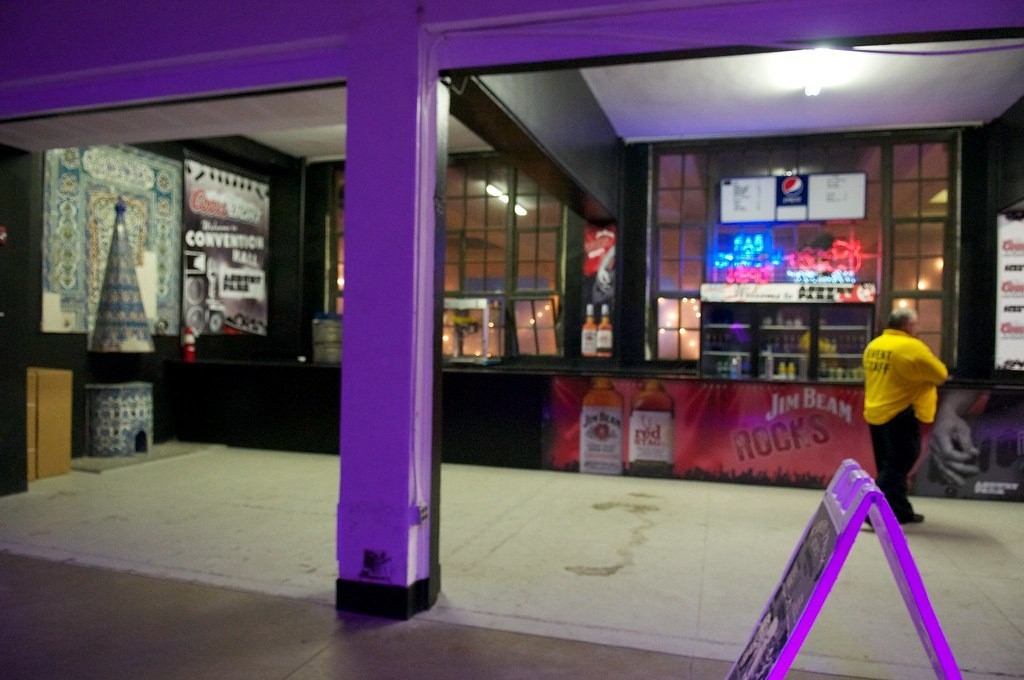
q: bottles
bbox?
[595,303,613,357]
[580,303,596,356]
[580,377,625,475]
[699,303,869,380]
[626,379,676,480]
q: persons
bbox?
[864,307,949,527]
[799,317,838,377]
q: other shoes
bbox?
[866,512,924,527]
